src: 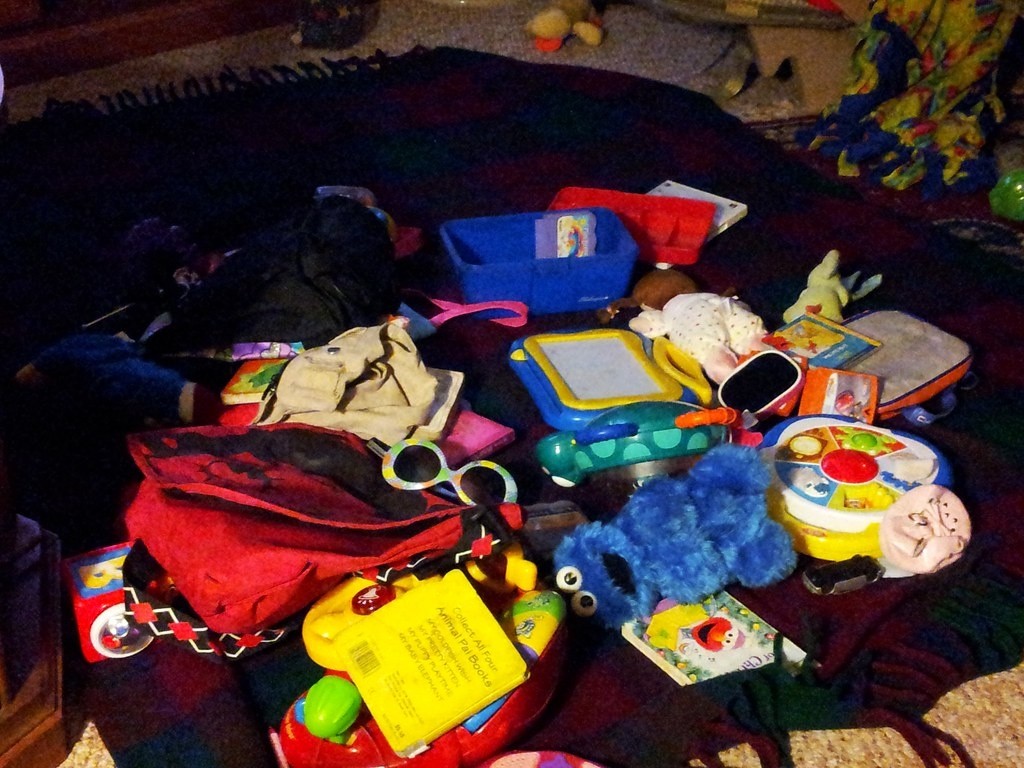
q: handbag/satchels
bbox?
[122,422,521,661]
[249,323,439,445]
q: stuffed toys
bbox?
[552,443,798,631]
[628,292,767,386]
[781,250,848,325]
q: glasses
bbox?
[381,438,517,508]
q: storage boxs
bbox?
[440,206,639,320]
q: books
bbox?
[621,587,823,688]
